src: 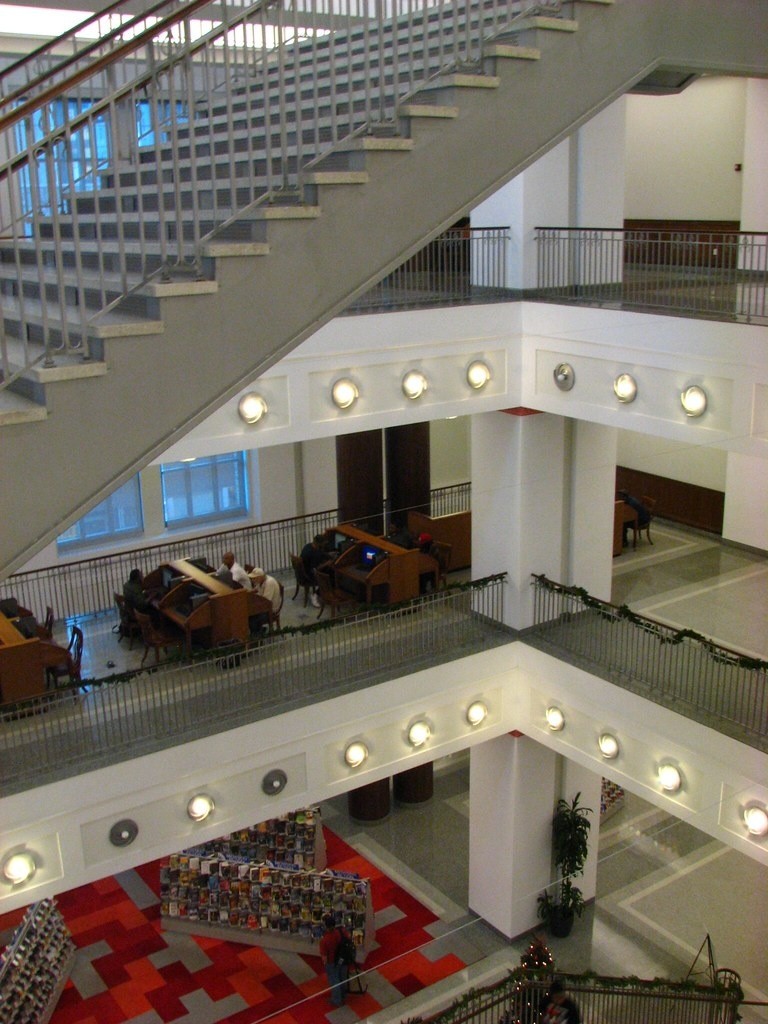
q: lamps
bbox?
[3,854,39,884]
[237,358,707,424]
[109,701,767,847]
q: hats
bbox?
[247,567,266,577]
[617,488,629,497]
[419,533,432,544]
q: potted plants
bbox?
[535,791,593,939]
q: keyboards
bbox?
[357,564,372,571]
[175,603,191,617]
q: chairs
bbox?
[629,496,657,549]
[25,513,463,706]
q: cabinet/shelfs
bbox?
[0,897,77,1024]
[162,806,376,960]
[601,778,626,827]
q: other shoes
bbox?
[310,593,322,608]
[624,542,628,546]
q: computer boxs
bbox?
[338,539,356,557]
[371,551,388,569]
[191,593,212,611]
[169,576,186,590]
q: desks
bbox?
[613,499,638,555]
[143,556,271,661]
[0,610,48,716]
[319,524,423,610]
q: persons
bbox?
[617,489,650,547]
[122,568,161,633]
[381,520,444,595]
[319,917,352,1006]
[215,551,282,637]
[301,534,338,607]
[539,980,582,1024]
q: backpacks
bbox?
[215,638,241,668]
[334,926,357,967]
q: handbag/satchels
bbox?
[112,623,133,637]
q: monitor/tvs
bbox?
[189,583,203,597]
[161,568,174,593]
[362,546,380,564]
[334,533,347,549]
